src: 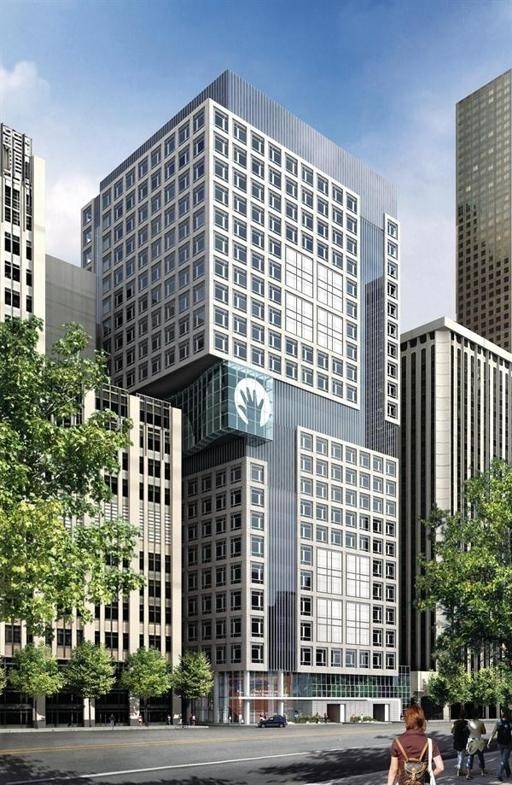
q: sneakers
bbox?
[455,768,489,780]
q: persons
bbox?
[387,704,445,785]
[486,707,512,782]
[465,711,487,781]
[451,711,470,777]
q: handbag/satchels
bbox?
[428,737,436,785]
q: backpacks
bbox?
[395,736,429,785]
[496,718,511,745]
